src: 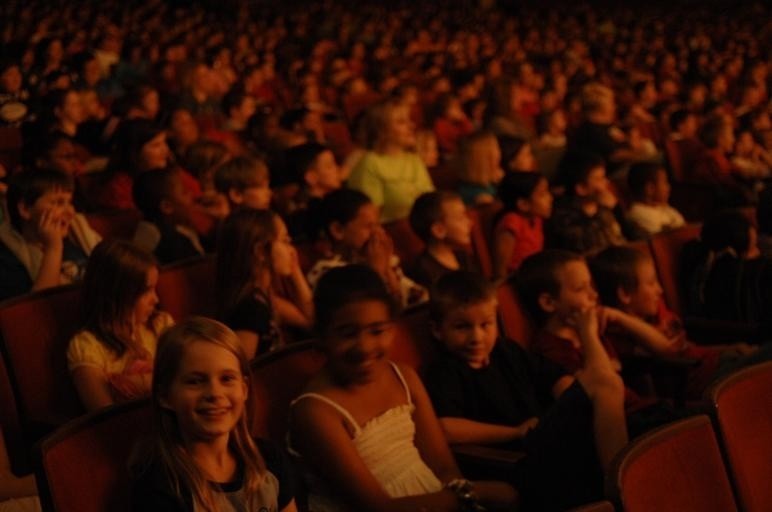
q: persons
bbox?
[1,1,771,511]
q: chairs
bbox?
[0,75,772,512]
